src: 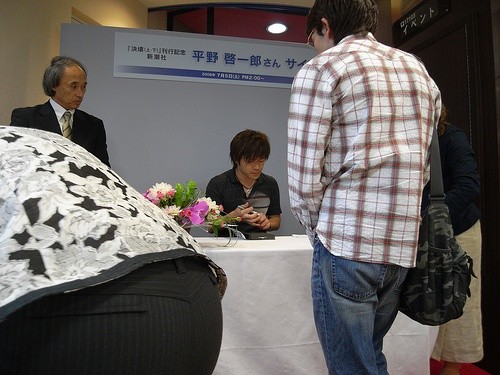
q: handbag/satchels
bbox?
[396,194,476,327]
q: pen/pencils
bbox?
[238,206,260,215]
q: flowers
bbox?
[142,182,238,233]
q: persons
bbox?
[10,56,111,168]
[287,0,442,375]
[421,104,482,375]
[205,129,283,237]
[0,126,227,375]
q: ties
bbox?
[62,111,73,140]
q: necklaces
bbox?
[242,184,252,192]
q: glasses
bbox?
[307,28,317,47]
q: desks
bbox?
[193,234,430,375]
